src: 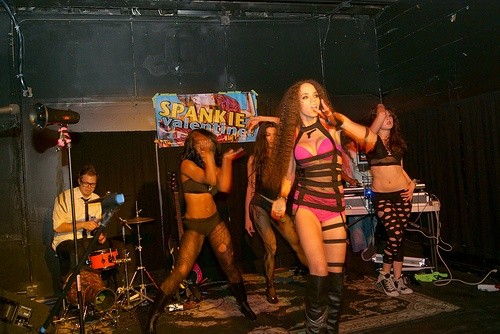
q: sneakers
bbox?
[379,271,399,297]
[392,274,413,294]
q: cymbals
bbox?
[83,194,133,203]
[119,217,153,224]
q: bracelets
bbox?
[277,195,288,201]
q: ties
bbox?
[81,197,90,250]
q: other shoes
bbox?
[265,283,277,303]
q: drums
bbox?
[63,271,116,310]
[87,247,119,270]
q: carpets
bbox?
[52,266,462,334]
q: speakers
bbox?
[0,288,58,334]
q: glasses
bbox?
[80,177,96,188]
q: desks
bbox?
[345,200,441,273]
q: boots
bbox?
[326,267,346,334]
[146,287,167,334]
[305,272,329,334]
[229,280,257,321]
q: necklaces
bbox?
[307,129,316,138]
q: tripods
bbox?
[119,223,159,307]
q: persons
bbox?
[52,164,136,299]
[314,103,416,296]
[262,80,377,334]
[143,128,257,334]
[245,116,310,304]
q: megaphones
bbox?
[28,102,80,130]
